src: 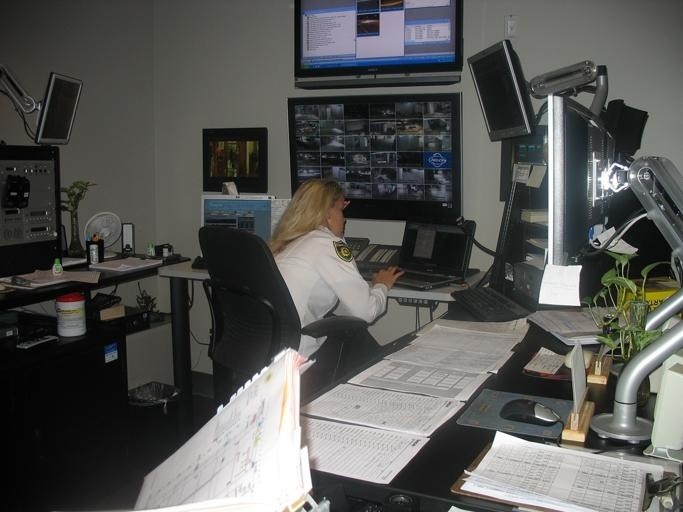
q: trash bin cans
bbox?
[126,382,183,450]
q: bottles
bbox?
[147,240,154,256]
[52,257,64,277]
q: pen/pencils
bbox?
[85,232,101,242]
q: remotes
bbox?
[17,336,58,349]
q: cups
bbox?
[86,239,104,264]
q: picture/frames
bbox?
[200,124,270,195]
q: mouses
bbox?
[501,398,561,426]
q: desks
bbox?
[153,249,488,424]
[0,248,194,456]
[276,296,663,510]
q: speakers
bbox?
[60,225,68,258]
[122,223,135,256]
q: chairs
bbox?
[194,224,371,426]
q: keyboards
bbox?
[450,287,526,322]
[192,256,209,269]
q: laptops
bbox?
[360,220,476,290]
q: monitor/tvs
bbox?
[294,0,463,89]
[201,195,275,241]
[35,72,83,145]
[288,93,462,224]
[467,39,535,141]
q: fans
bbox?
[80,209,125,259]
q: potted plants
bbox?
[56,178,100,259]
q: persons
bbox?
[263,179,405,401]
